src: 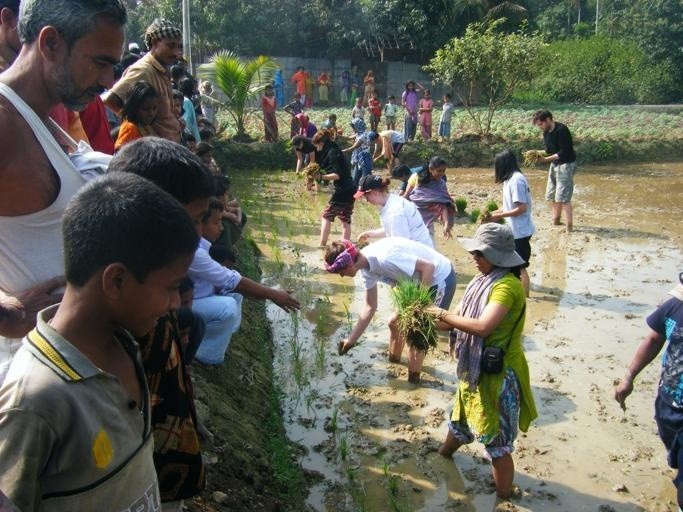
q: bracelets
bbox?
[438,309,443,322]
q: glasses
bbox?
[468,250,484,257]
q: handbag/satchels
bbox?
[482,345,504,373]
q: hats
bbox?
[353,175,383,199]
[457,222,526,268]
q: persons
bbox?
[487,149,536,300]
[524,104,577,234]
[324,234,456,383]
[260,65,301,176]
[300,64,459,250]
[0,0,249,510]
[613,270,683,512]
[416,219,540,501]
[176,197,301,366]
